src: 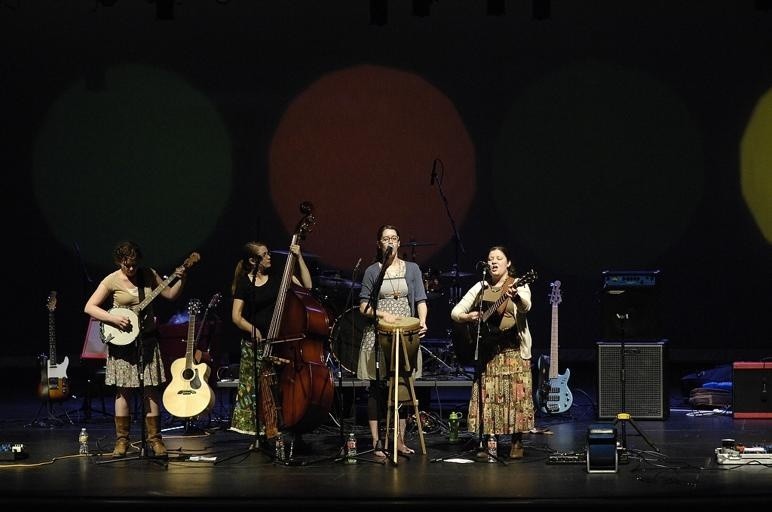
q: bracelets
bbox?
[513,294,520,304]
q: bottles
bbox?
[275,432,295,460]
[346,431,359,464]
[78,427,89,454]
[721,437,768,454]
[486,433,498,463]
[447,411,463,444]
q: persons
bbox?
[356,226,428,457]
[451,246,535,458]
[83,241,188,458]
[230,240,313,456]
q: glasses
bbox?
[380,234,399,245]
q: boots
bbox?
[110,413,134,458]
[143,412,168,459]
[508,432,525,460]
[476,434,499,458]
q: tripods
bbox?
[430,271,508,466]
[301,273,386,466]
[26,354,63,429]
[333,255,411,467]
[214,263,292,465]
[424,183,474,380]
[611,312,660,466]
[95,334,190,464]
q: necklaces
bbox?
[489,275,510,293]
[382,260,401,299]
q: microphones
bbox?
[477,262,492,271]
[384,244,394,255]
[760,380,768,401]
[353,257,361,272]
[430,160,437,185]
[250,254,263,263]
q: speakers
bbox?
[596,341,670,421]
[732,361,772,419]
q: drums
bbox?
[377,315,420,401]
[330,308,367,374]
[420,274,444,299]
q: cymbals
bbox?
[439,272,472,277]
[270,251,320,257]
[400,242,438,246]
[316,276,363,289]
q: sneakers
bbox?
[396,444,416,455]
[372,447,389,457]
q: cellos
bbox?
[257,202,335,433]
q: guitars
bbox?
[463,268,537,362]
[536,280,572,415]
[161,299,215,420]
[37,292,71,401]
[194,292,222,363]
